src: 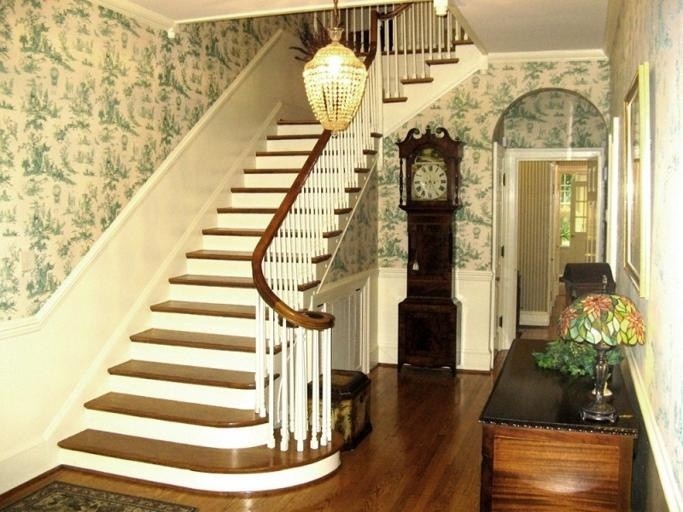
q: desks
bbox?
[478,337,640,512]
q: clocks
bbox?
[407,161,448,201]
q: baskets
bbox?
[558,262,616,308]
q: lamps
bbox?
[302,1,368,135]
[557,295,644,424]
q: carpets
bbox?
[1,482,196,512]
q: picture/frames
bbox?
[598,63,651,289]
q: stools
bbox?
[308,369,372,455]
